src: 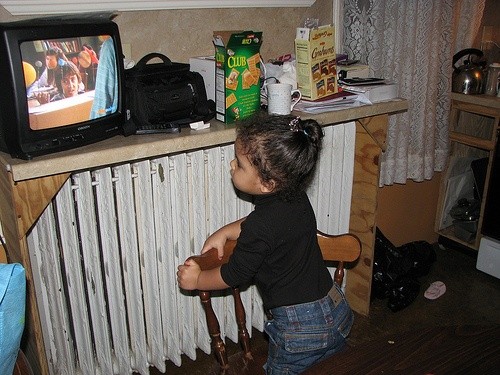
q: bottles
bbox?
[485,62,500,97]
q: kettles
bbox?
[452,48,489,96]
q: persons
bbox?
[90,35,118,119]
[28,47,64,98]
[177,113,354,375]
[51,61,85,102]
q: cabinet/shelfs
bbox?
[433,92,500,258]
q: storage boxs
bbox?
[343,84,401,104]
[475,237,500,278]
[211,31,263,124]
[338,64,370,78]
[296,24,338,102]
[189,56,215,101]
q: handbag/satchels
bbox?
[122,52,216,136]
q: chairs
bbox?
[184,231,362,375]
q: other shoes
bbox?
[425,281,447,300]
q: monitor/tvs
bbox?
[0,18,127,161]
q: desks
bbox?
[0,98,409,375]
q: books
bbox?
[43,41,78,54]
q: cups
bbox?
[266,83,302,115]
